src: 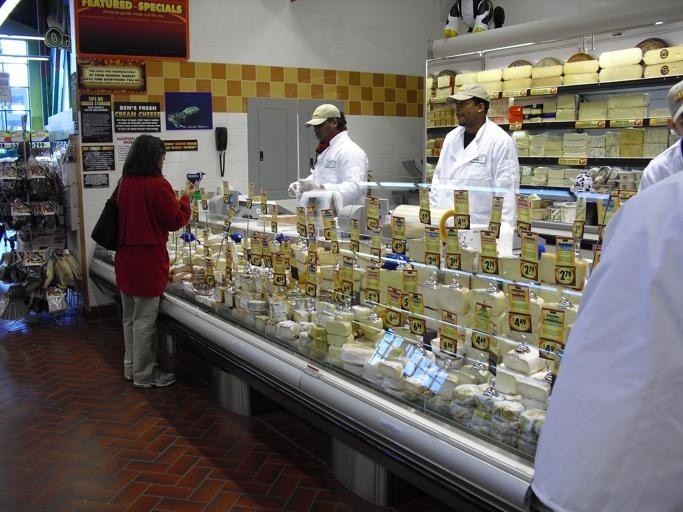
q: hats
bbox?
[667,80,683,122]
[304,104,341,128]
[445,82,491,105]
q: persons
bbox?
[429,83,519,229]
[115,134,197,388]
[287,104,369,212]
[528,170,683,512]
[637,80,683,193]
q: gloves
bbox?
[299,180,314,191]
[287,182,296,198]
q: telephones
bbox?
[215,127,227,151]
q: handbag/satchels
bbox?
[90,199,119,251]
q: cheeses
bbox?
[166,195,623,456]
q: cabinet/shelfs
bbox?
[424,18,683,268]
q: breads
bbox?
[426,45,683,188]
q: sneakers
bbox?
[134,372,176,388]
